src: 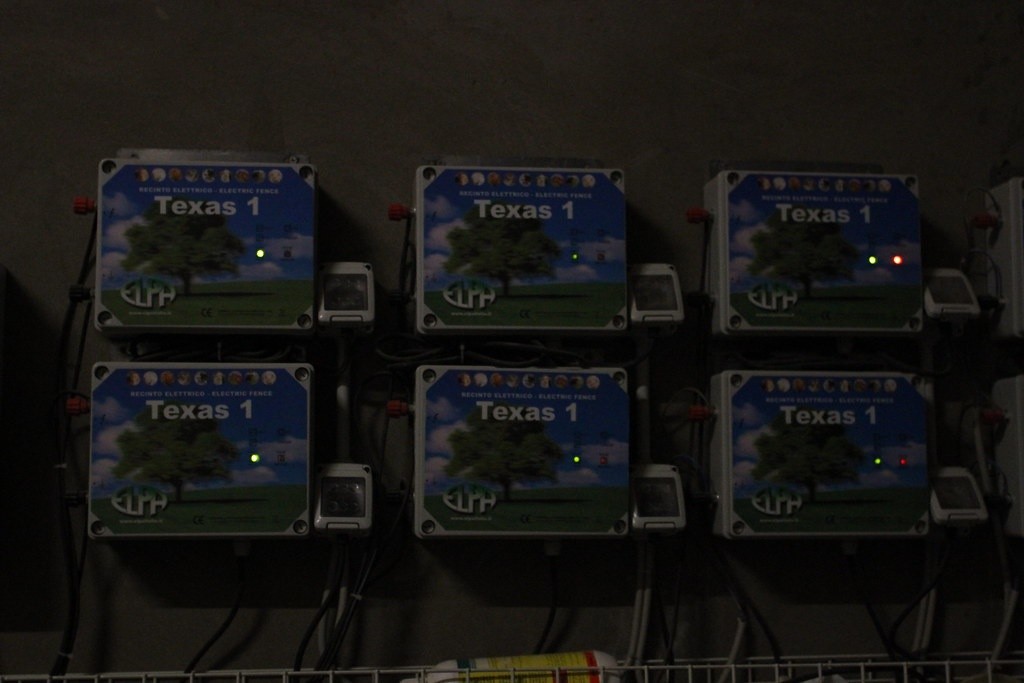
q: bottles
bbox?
[396,651,622,683]
